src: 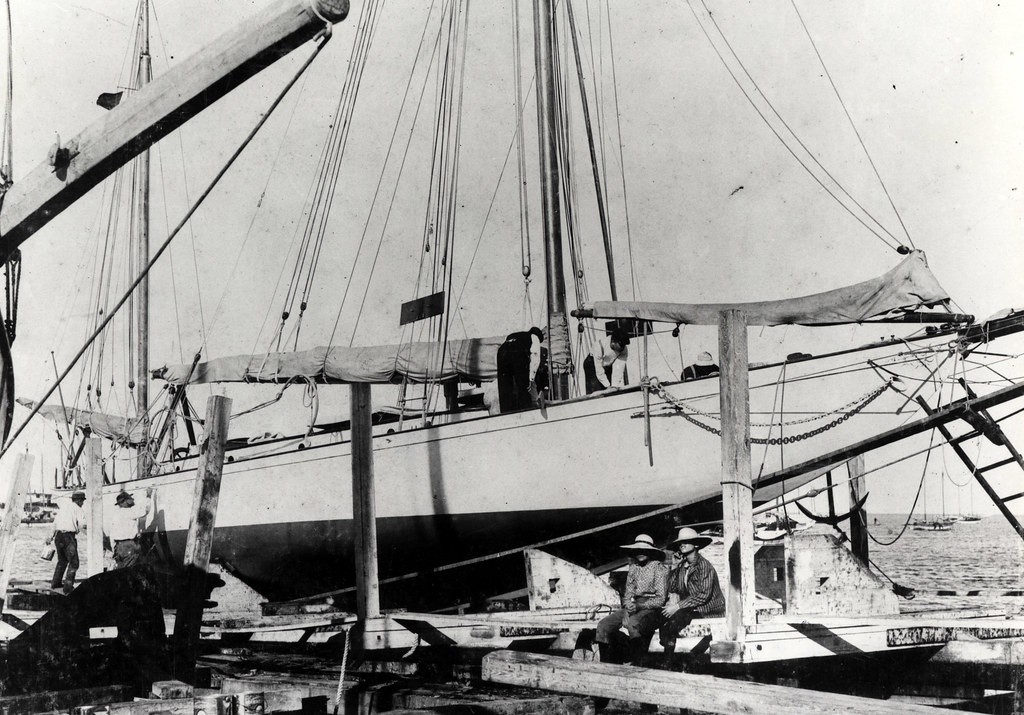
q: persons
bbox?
[596,534,668,668]
[681,352,719,380]
[583,328,630,395]
[46,491,87,594]
[659,527,726,670]
[109,487,152,570]
[496,327,544,414]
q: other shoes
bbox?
[52,583,64,588]
[64,587,74,593]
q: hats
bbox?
[67,491,86,501]
[619,534,666,564]
[114,492,133,505]
[667,527,713,554]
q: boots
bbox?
[658,643,675,669]
[632,644,648,667]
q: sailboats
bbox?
[2,0,1024,714]
